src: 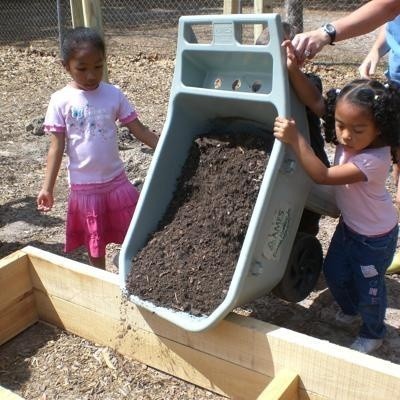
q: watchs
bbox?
[321,23,336,46]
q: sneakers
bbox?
[352,337,384,354]
[331,311,362,330]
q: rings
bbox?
[303,50,311,56]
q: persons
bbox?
[33,25,160,270]
[288,1,400,277]
[270,38,400,356]
